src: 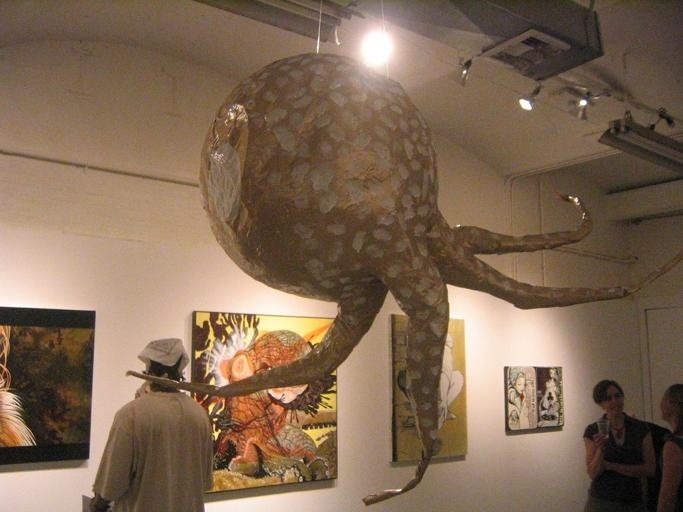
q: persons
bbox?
[508,366,561,429]
[80,338,216,511]
[581,379,657,512]
[658,383,682,511]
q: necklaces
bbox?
[611,424,624,439]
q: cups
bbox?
[597,419,610,439]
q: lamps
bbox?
[444,48,592,121]
